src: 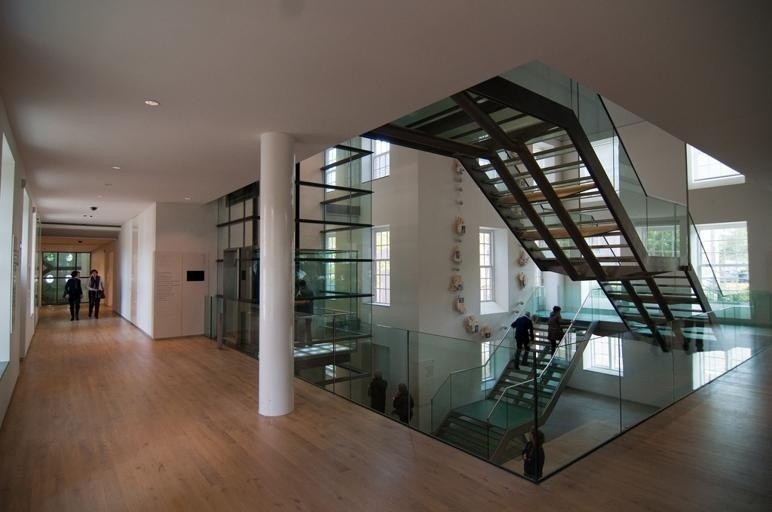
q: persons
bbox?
[521,429,545,481]
[64,270,84,321]
[87,268,105,319]
[547,306,576,358]
[392,382,415,424]
[511,311,534,370]
[365,369,388,415]
[294,278,315,348]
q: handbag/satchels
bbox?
[95,290,105,299]
